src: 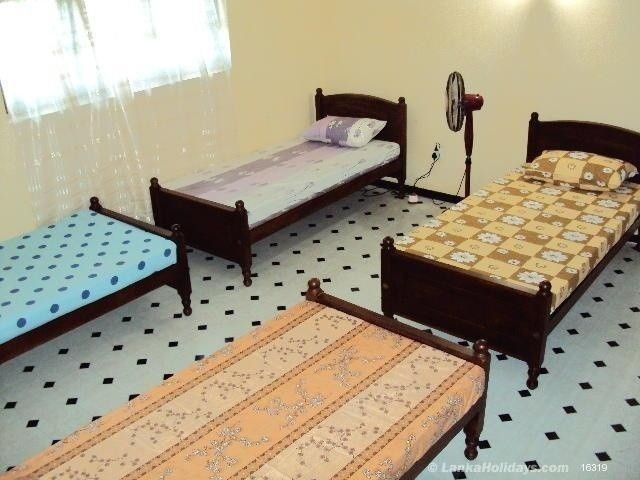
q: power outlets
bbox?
[431,148,442,160]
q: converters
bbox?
[408,195,418,202]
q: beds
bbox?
[148,89,408,287]
[0,275,491,480]
[381,112,639,389]
[0,189,193,364]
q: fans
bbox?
[445,69,484,197]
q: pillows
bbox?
[523,149,639,199]
[301,114,388,147]
